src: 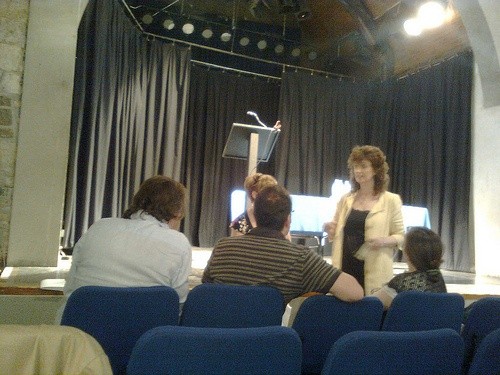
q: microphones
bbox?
[247,110,267,127]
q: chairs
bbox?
[0,324,114,375]
[126,326,302,375]
[180,283,285,327]
[60,285,179,375]
[291,294,384,375]
[384,295,500,375]
[321,328,464,375]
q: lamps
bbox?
[142,0,332,62]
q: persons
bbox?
[322,145,405,296]
[201,185,364,316]
[229,173,292,241]
[54,176,191,324]
[372,226,448,312]
